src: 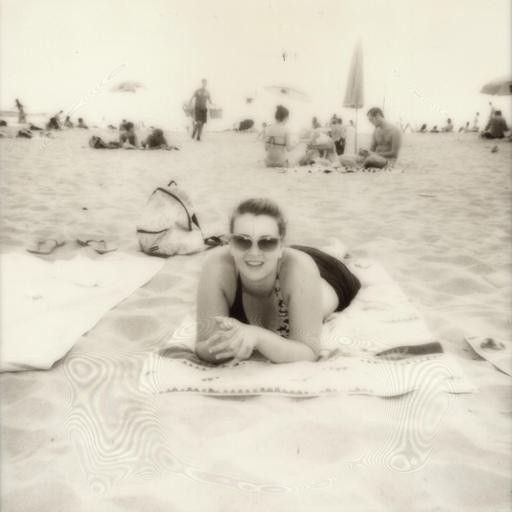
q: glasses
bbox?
[231,235,279,253]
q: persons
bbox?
[1,99,86,138]
[262,104,400,171]
[191,79,211,140]
[405,112,478,134]
[89,120,168,148]
[200,197,361,368]
[481,100,508,140]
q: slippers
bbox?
[77,234,116,253]
[27,234,66,254]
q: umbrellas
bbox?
[343,39,368,150]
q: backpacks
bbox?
[137,181,221,256]
